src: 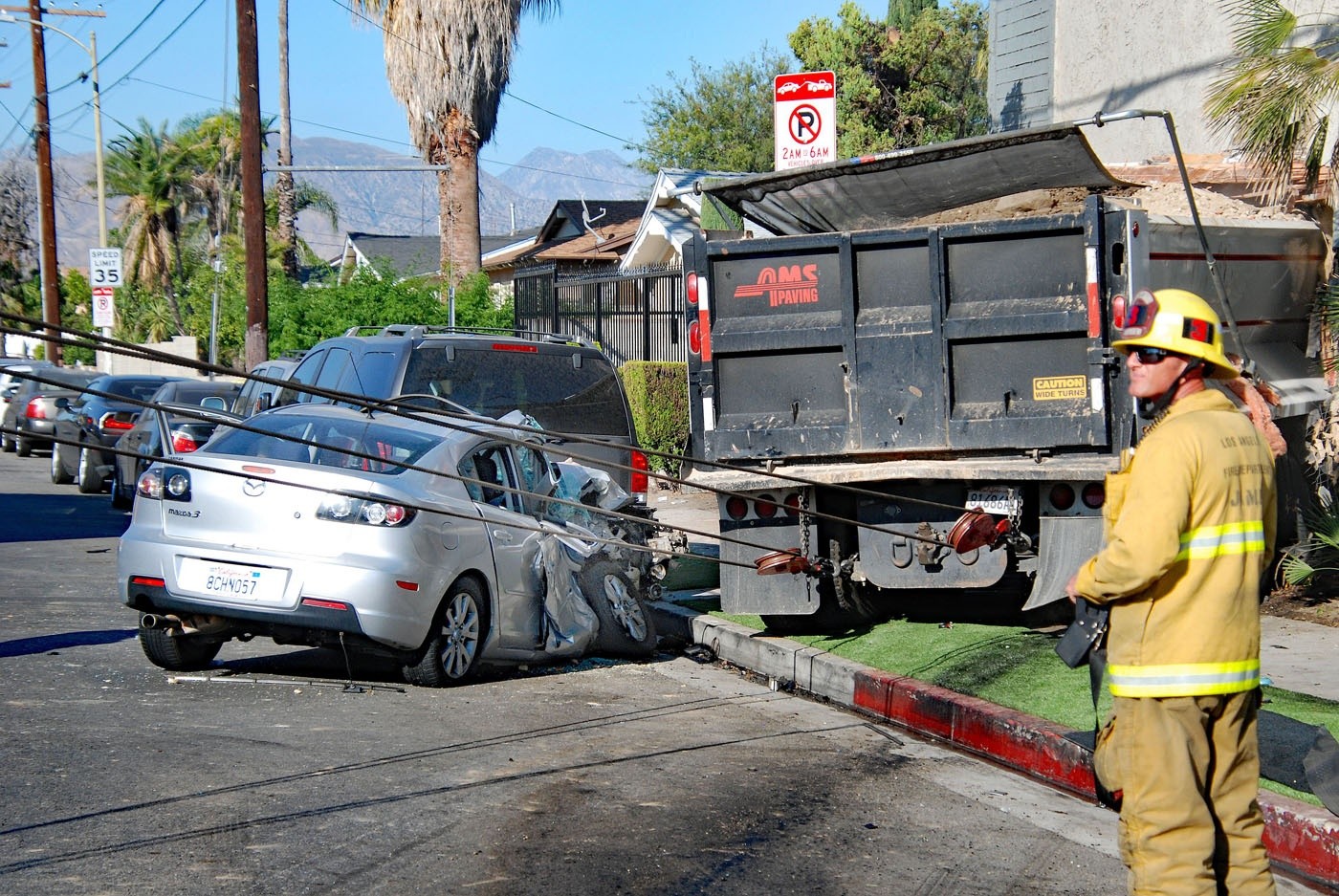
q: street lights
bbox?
[0,13,114,374]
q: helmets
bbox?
[1112,289,1240,379]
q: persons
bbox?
[1065,285,1278,896]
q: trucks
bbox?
[668,108,1339,633]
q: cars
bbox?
[0,355,57,448]
[116,392,692,690]
[111,379,246,511]
[0,367,112,457]
[50,373,203,494]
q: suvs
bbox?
[255,324,651,507]
[200,348,311,442]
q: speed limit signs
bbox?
[88,247,124,287]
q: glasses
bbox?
[1125,345,1191,364]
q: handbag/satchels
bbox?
[1055,596,1109,669]
